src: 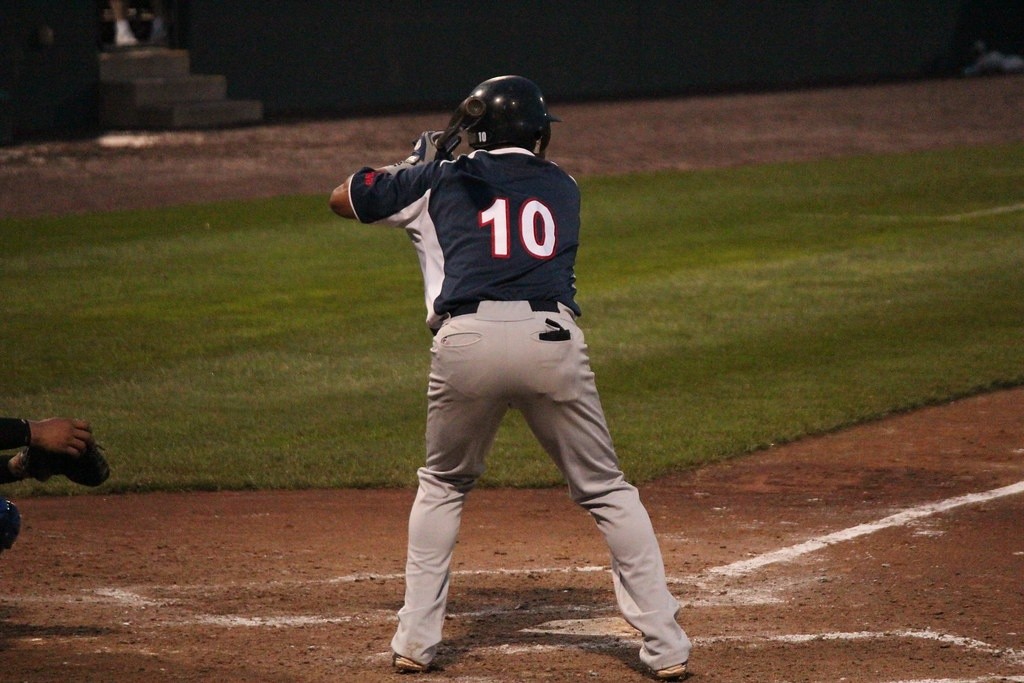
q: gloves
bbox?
[383,130,454,179]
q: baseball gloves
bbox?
[18,438,113,490]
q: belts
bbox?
[450,300,559,319]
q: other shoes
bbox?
[648,660,687,679]
[395,655,428,670]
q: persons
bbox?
[0,416,92,552]
[330,77,691,676]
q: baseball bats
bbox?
[434,95,486,149]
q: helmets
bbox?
[467,75,562,154]
